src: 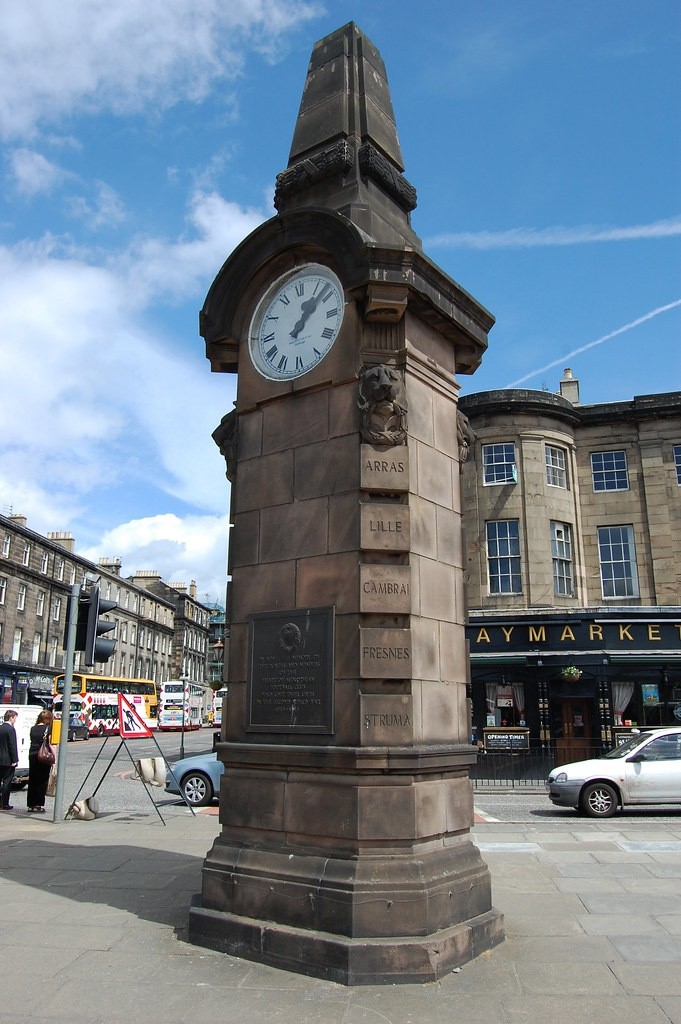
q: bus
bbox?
[156,680,206,732]
[210,686,228,728]
[50,673,158,736]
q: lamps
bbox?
[212,638,224,660]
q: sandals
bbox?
[32,805,45,812]
[26,806,32,811]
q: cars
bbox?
[163,750,224,807]
[547,726,681,818]
[67,717,90,742]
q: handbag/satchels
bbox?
[46,765,56,797]
[38,726,55,764]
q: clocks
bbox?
[247,262,345,381]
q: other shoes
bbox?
[0,805,13,809]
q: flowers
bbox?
[560,666,583,676]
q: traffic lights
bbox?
[85,584,118,667]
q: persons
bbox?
[27,710,55,812]
[0,710,19,810]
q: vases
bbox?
[562,675,579,683]
